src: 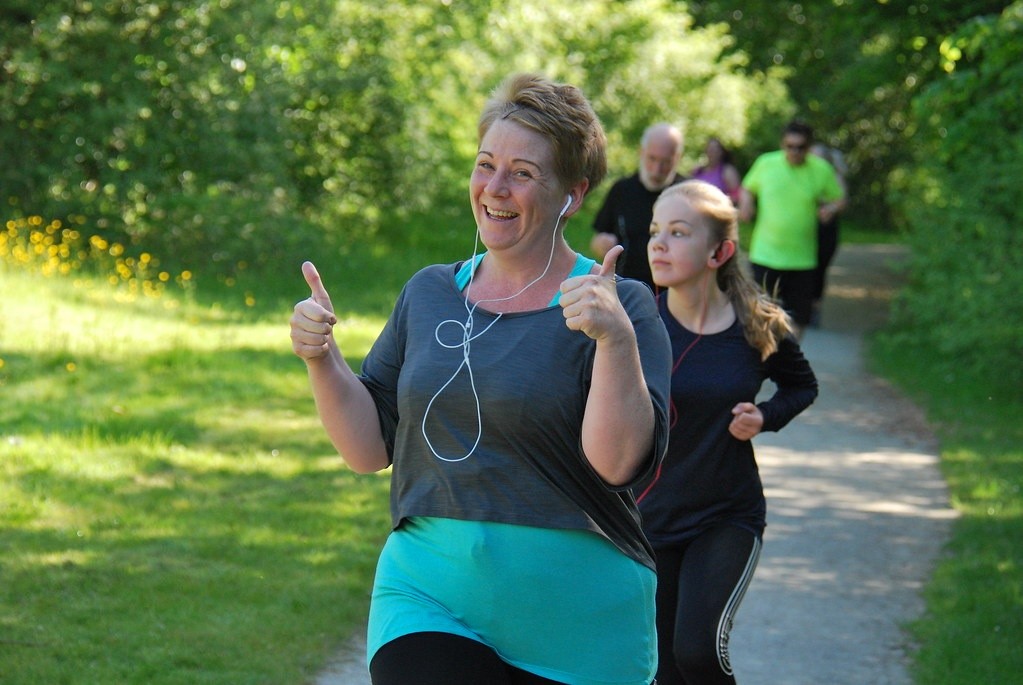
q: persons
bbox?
[736,122,846,344]
[809,140,845,298]
[288,70,675,684]
[586,119,690,296]
[629,179,819,685]
[690,137,741,203]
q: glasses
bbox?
[784,142,806,152]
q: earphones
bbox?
[712,246,723,262]
[559,194,572,216]
